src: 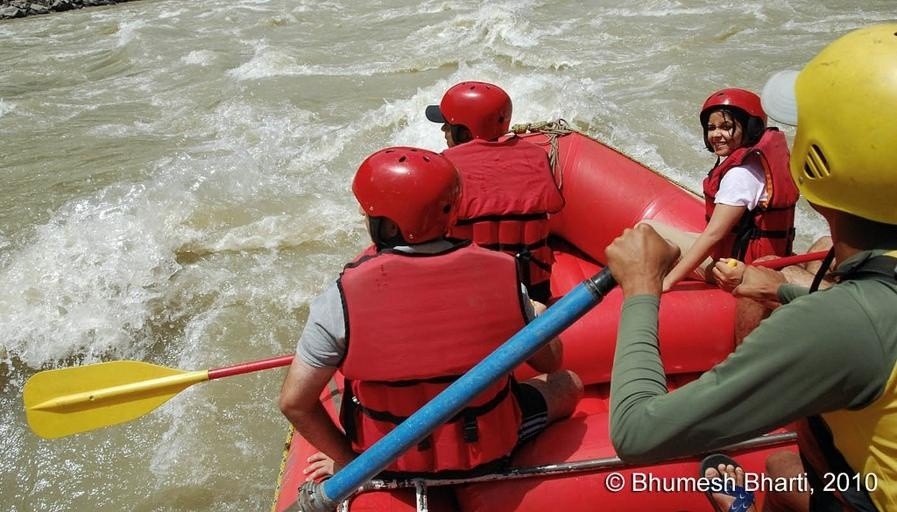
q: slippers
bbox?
[701,454,756,512]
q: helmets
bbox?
[352,147,461,246]
[788,24,896,224]
[440,82,513,141]
[700,88,770,153]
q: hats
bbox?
[426,105,444,123]
[761,68,801,129]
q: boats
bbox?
[271,120,806,512]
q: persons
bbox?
[657,87,794,293]
[274,142,589,486]
[602,21,896,511]
[423,81,556,299]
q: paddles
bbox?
[22,357,294,439]
